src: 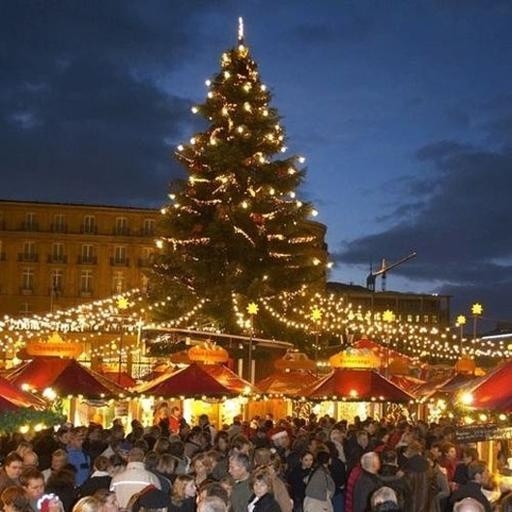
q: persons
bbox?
[1,404,512,511]
